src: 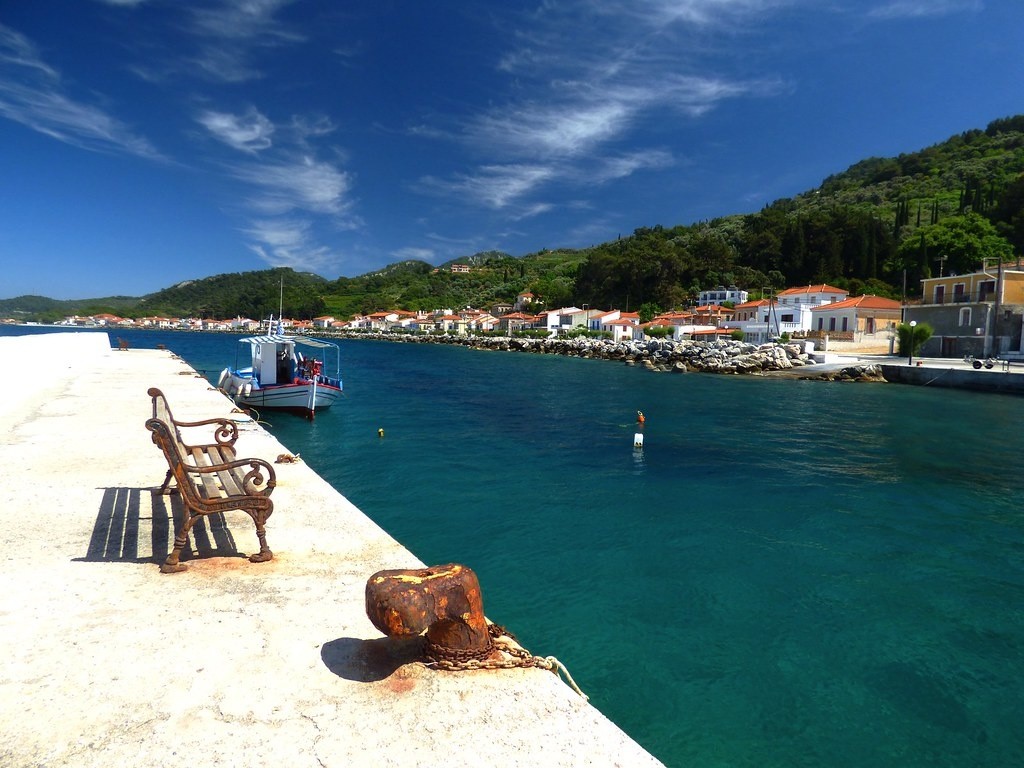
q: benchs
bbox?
[144,388,276,574]
[1002,358,1024,371]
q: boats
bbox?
[764,286,805,348]
[296,315,315,338]
[217,270,343,419]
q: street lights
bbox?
[582,304,588,338]
[535,330,538,338]
[908,321,917,363]
[588,329,590,339]
[725,325,728,338]
[650,326,653,339]
[505,330,507,336]
